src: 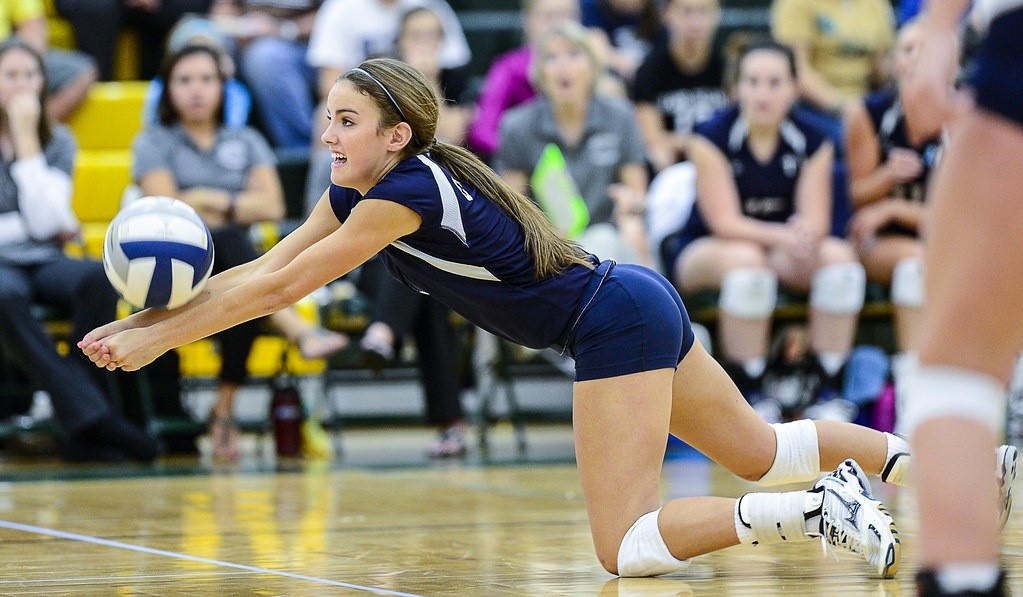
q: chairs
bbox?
[122,279,563,467]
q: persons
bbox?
[76,58,1018,580]
[894,0,1023,597]
[0,0,941,467]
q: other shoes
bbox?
[363,338,393,365]
[208,407,238,465]
[61,418,161,464]
[296,328,349,359]
[425,427,467,459]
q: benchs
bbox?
[691,283,890,324]
[49,77,323,386]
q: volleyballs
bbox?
[100,193,216,312]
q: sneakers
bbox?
[803,458,901,579]
[990,444,1018,543]
